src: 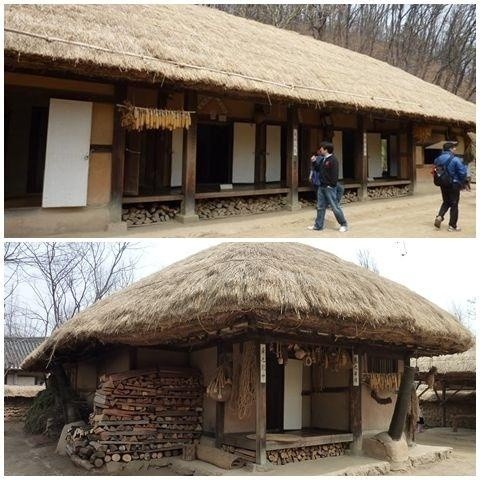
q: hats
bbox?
[443,142,458,152]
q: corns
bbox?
[370,372,402,393]
[131,107,192,132]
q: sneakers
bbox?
[434,216,442,228]
[447,225,460,231]
[307,225,324,230]
[339,226,348,232]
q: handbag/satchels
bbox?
[434,165,453,187]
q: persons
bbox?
[417,404,425,433]
[433,143,467,232]
[310,146,345,211]
[308,143,348,232]
[452,413,458,433]
